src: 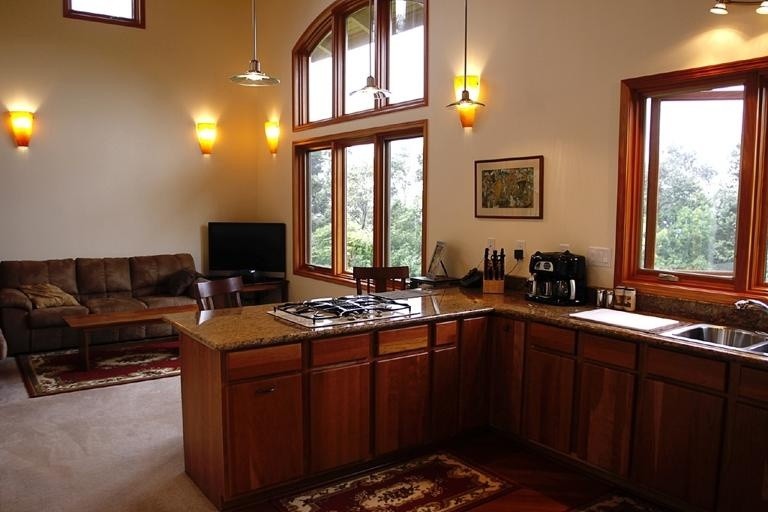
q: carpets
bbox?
[14,315,211,396]
[288,455,519,512]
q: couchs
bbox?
[0,250,206,354]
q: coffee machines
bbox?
[524,248,588,305]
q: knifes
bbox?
[481,246,505,280]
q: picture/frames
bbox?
[474,155,545,218]
[425,240,450,281]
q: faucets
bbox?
[735,299,768,315]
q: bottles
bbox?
[613,286,637,313]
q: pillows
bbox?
[18,281,80,309]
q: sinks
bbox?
[748,343,768,356]
[672,324,768,351]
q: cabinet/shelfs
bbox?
[636,336,768,512]
[367,316,502,461]
[519,315,636,488]
[309,334,378,479]
[180,338,308,512]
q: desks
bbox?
[235,279,290,309]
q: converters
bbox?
[514,250,523,259]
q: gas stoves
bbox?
[266,293,412,328]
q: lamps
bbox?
[350,0,391,99]
[264,119,282,155]
[7,109,34,149]
[228,1,280,91]
[446,1,483,113]
[709,1,768,15]
[453,73,483,127]
[195,121,219,155]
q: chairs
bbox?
[353,264,411,295]
[192,274,245,310]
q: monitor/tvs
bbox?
[208,222,286,285]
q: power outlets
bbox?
[513,240,527,259]
